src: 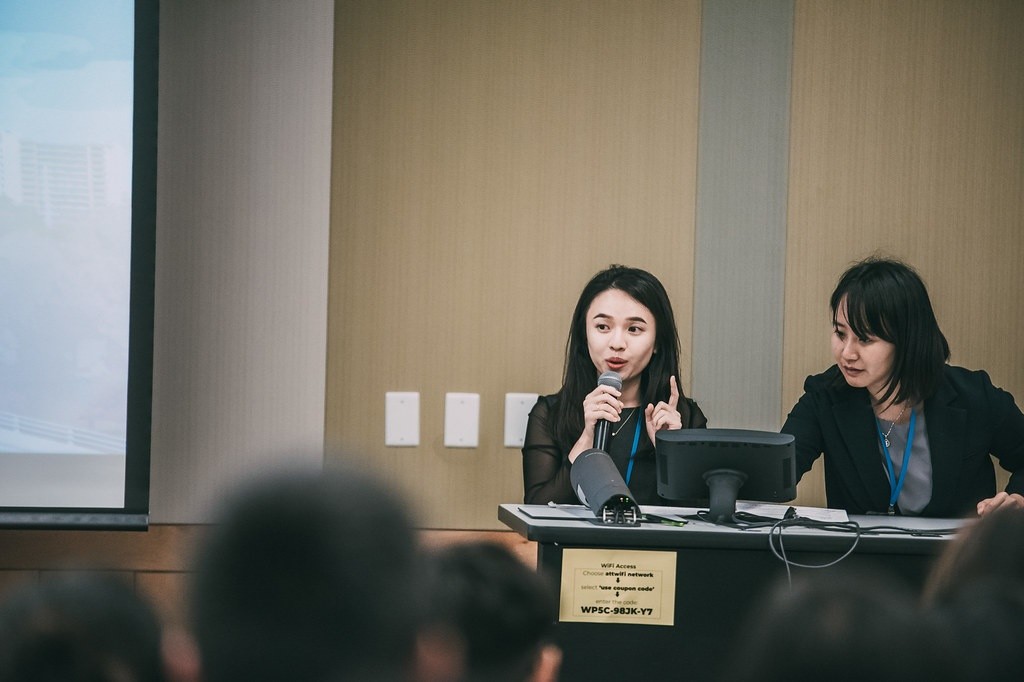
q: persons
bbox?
[782,254,1022,514]
[523,265,709,506]
[0,448,562,682]
[718,514,1023,682]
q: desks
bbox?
[497,504,984,682]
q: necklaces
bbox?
[880,400,909,447]
[609,400,641,436]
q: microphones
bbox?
[592,371,623,452]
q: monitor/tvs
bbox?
[656,429,797,503]
